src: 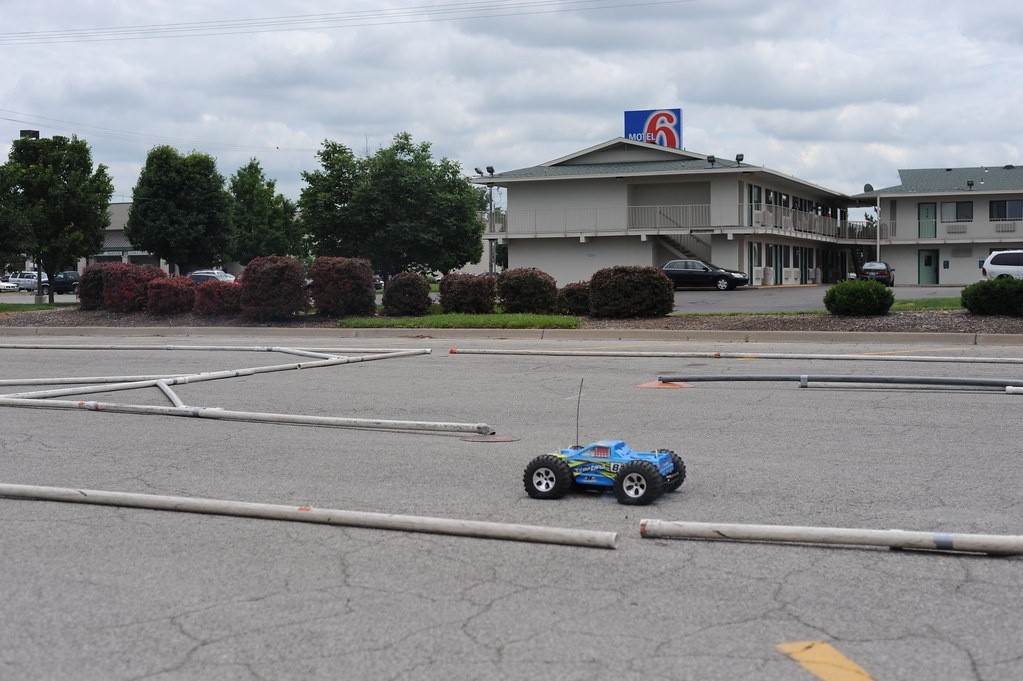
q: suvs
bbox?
[522,438,687,505]
[37,270,81,295]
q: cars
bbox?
[184,269,235,284]
[857,261,896,287]
[0,275,19,292]
[654,258,750,290]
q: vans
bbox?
[981,249,1023,279]
[8,271,49,290]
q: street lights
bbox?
[474,165,495,279]
